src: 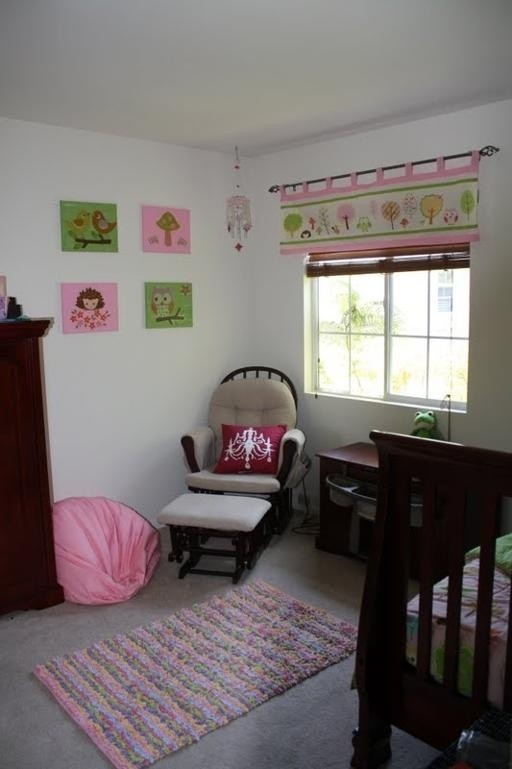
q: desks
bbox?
[313,441,424,582]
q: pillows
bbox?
[210,422,287,476]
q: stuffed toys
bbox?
[411,410,442,440]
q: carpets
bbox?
[32,573,359,769]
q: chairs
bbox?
[181,366,306,536]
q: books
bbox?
[0,276,8,320]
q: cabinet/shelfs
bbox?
[0,318,65,617]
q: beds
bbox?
[354,430,512,768]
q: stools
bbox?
[155,494,271,583]
